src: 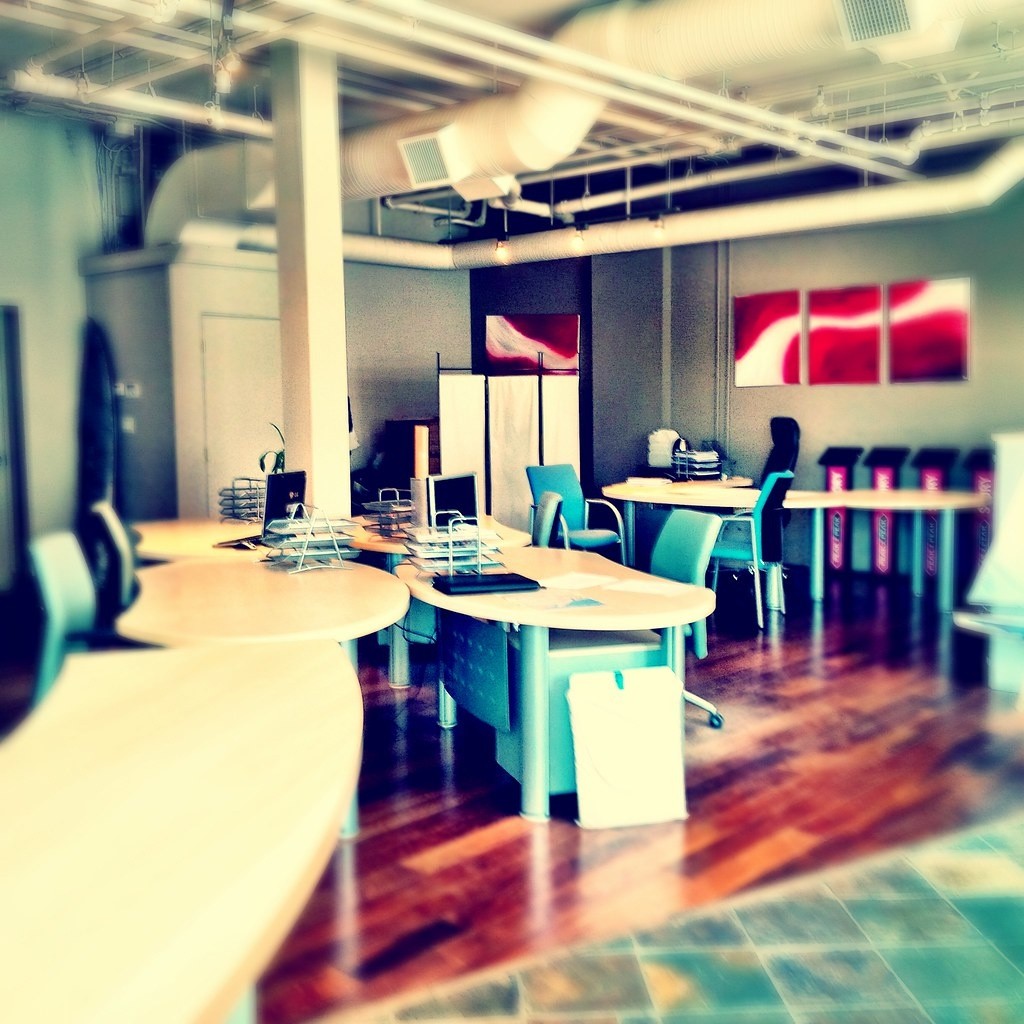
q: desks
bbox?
[600,476,987,614]
[339,514,532,554]
[395,550,717,823]
[132,515,356,562]
[114,557,412,648]
[0,641,365,1024]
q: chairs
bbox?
[28,528,149,710]
[525,416,801,729]
[91,500,138,640]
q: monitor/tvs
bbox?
[425,472,477,528]
[263,471,306,540]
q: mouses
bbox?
[234,542,257,550]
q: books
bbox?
[432,572,545,595]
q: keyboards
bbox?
[215,535,263,547]
